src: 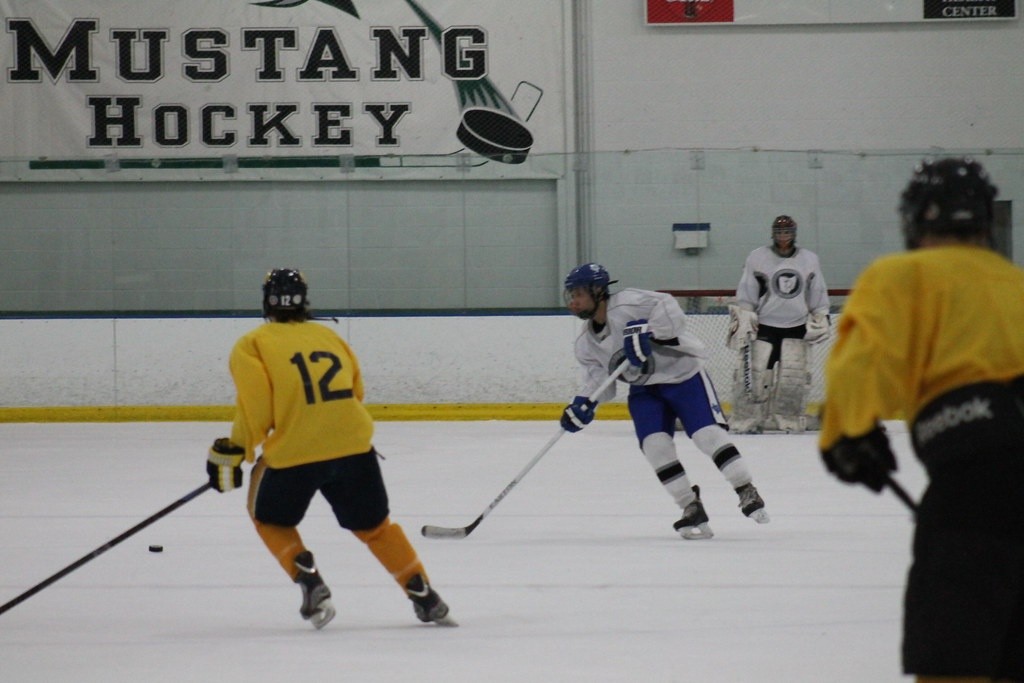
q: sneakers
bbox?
[673,486,714,540]
[734,482,768,523]
[405,576,458,626]
[290,551,337,628]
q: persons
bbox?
[563,260,765,528]
[205,268,448,625]
[819,157,1024,681]
[726,212,832,440]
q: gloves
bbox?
[825,429,898,493]
[207,437,245,492]
[561,397,595,432]
[623,319,654,366]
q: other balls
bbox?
[148,545,164,552]
[455,106,533,164]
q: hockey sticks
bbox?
[420,356,631,540]
[29,81,544,171]
[884,474,921,512]
[0,480,214,615]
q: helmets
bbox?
[772,216,797,230]
[262,269,307,315]
[902,156,997,235]
[564,262,610,288]
